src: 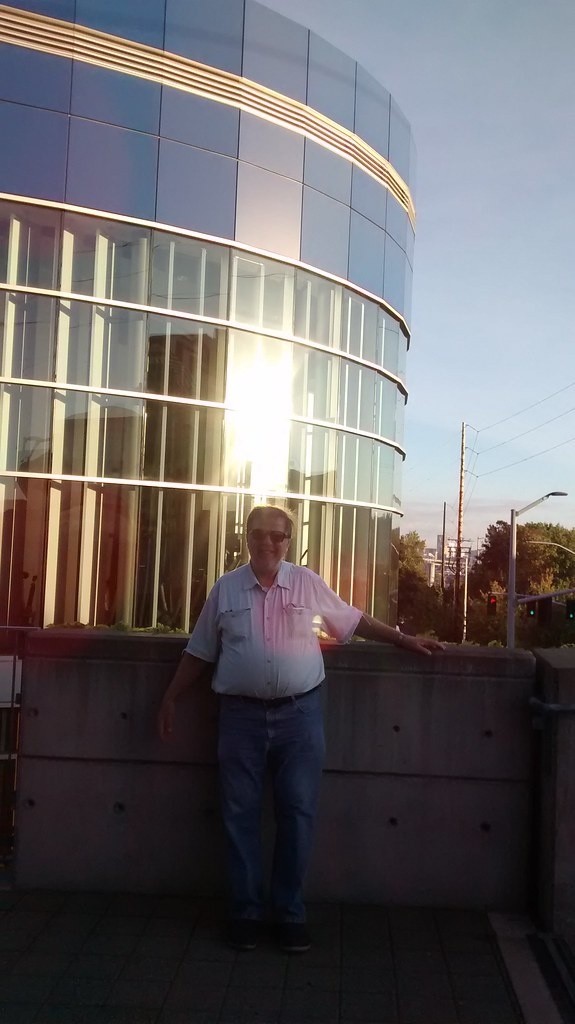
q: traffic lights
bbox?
[566,600,575,620]
[526,600,536,617]
[487,596,497,616]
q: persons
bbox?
[157,507,446,952]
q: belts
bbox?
[220,684,321,705]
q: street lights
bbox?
[507,492,568,648]
[446,555,468,641]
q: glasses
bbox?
[247,528,291,543]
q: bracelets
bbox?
[396,633,404,647]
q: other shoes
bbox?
[226,919,257,949]
[276,921,313,952]
